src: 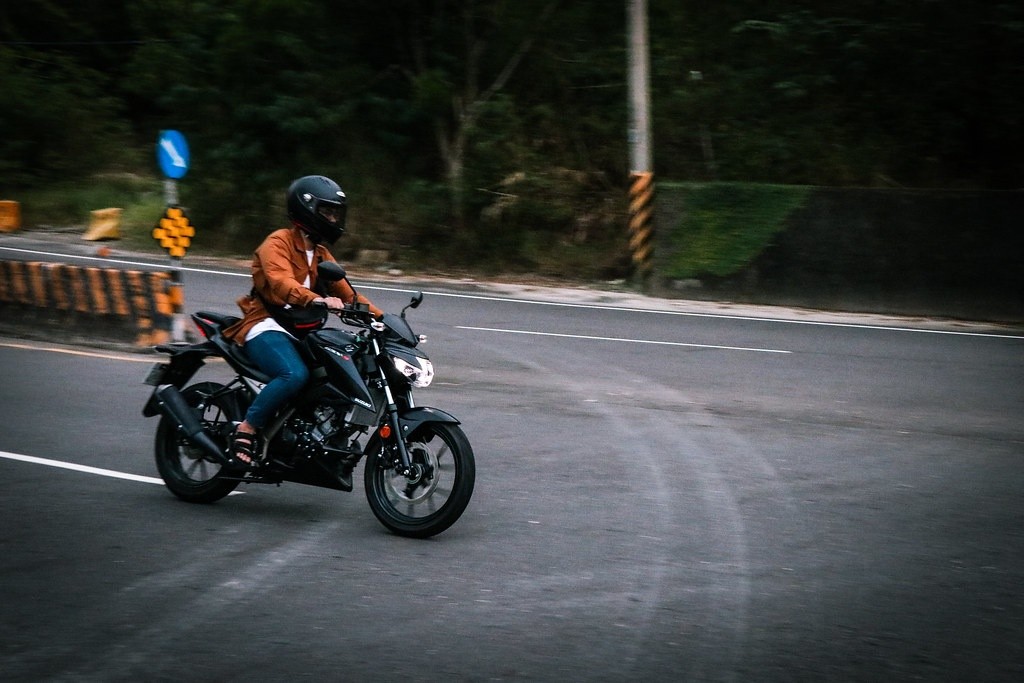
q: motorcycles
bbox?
[142,261,476,539]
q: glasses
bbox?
[319,206,340,219]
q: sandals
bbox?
[228,424,254,471]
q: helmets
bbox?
[287,175,347,245]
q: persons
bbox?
[220,176,386,474]
